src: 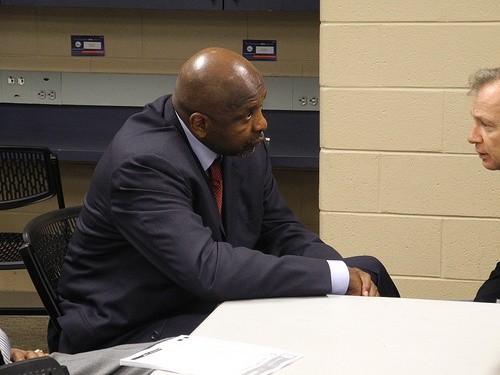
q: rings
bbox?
[34,349,42,354]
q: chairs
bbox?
[20,206,89,335]
[0,146,75,353]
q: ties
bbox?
[210,162,222,218]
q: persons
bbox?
[57,47,401,355]
[468,67,500,303]
[0,329,176,375]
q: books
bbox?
[120,335,307,375]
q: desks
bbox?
[152,295,500,375]
